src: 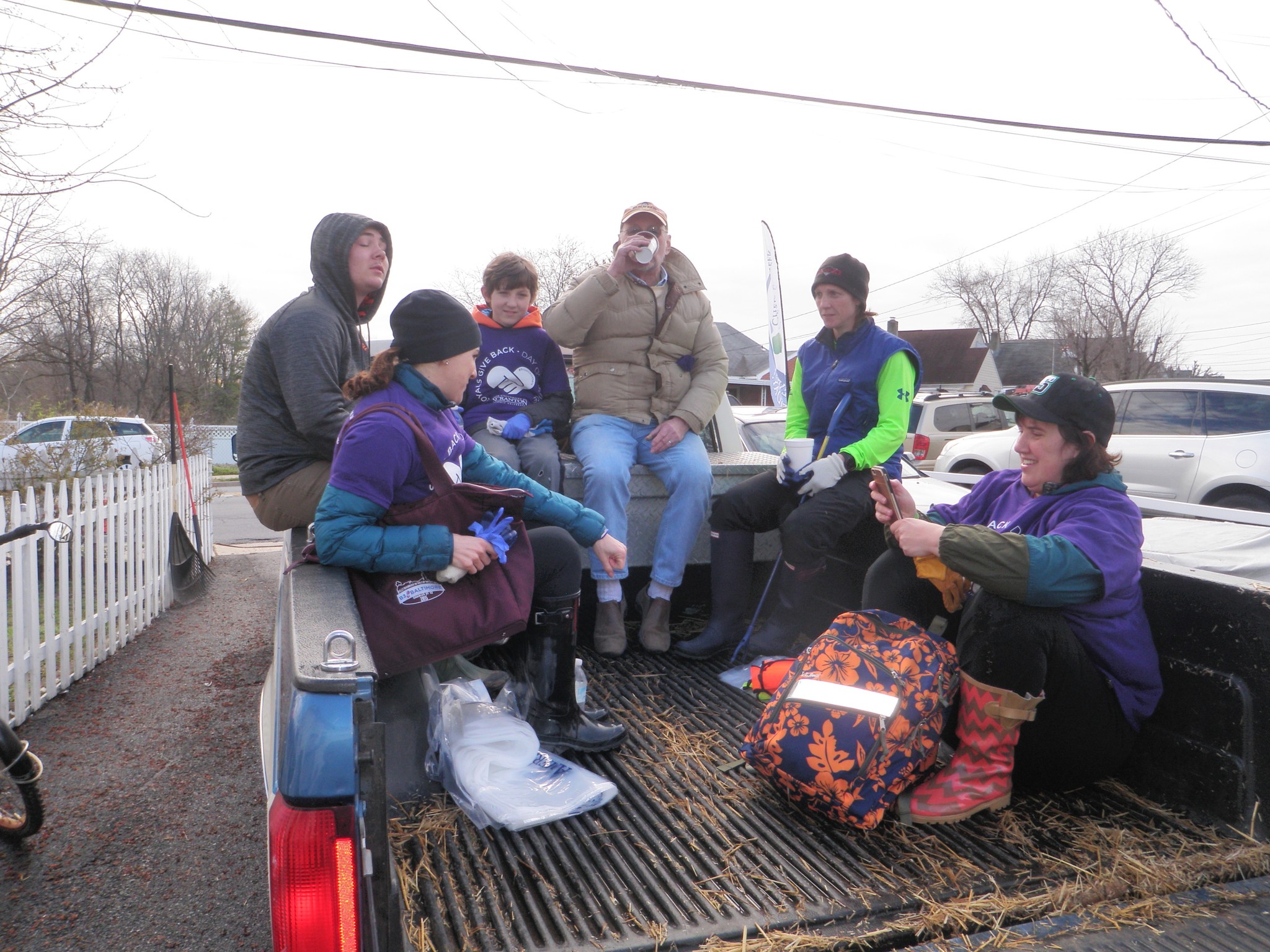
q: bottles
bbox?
[574,658,587,710]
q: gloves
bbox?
[776,453,795,491]
[487,417,554,438]
[436,508,517,583]
[793,452,848,497]
[501,413,531,440]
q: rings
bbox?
[668,440,672,444]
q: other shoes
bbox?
[634,581,671,653]
[593,592,627,661]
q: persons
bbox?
[858,369,1164,825]
[458,253,574,532]
[540,199,729,657]
[235,211,485,662]
[673,252,922,662]
[313,286,632,758]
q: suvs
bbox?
[901,389,1018,471]
[934,377,1270,528]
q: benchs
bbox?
[557,449,785,568]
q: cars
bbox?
[725,390,974,517]
[0,415,167,492]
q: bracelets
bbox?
[600,526,609,540]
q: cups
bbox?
[784,438,815,480]
[634,231,659,264]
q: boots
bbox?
[674,529,754,660]
[743,557,827,658]
[522,588,630,753]
[895,669,1045,824]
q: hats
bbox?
[390,289,482,363]
[620,202,667,228]
[811,253,870,309]
[992,372,1115,448]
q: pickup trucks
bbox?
[258,351,1267,951]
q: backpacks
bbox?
[738,609,961,830]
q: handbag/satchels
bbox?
[283,402,535,680]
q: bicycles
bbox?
[0,719,49,844]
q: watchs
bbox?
[839,452,856,471]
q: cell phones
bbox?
[871,466,903,524]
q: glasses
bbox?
[620,226,668,238]
